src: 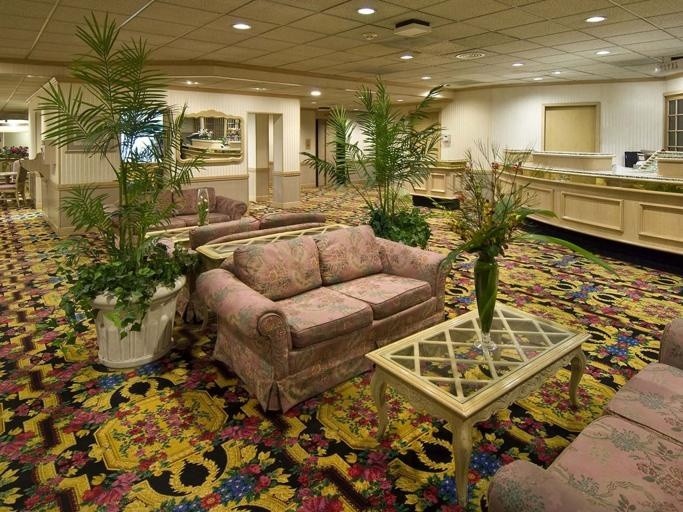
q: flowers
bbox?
[0,145,30,160]
[198,195,208,225]
[421,138,622,282]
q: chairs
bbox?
[0,157,27,208]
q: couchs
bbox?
[487,316,683,512]
[195,223,453,414]
[174,210,326,295]
[100,186,247,247]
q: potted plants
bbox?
[31,7,213,372]
[299,72,449,253]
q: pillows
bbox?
[234,225,384,301]
[141,187,216,218]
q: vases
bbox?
[474,252,498,351]
[197,189,209,225]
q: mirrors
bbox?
[175,109,245,164]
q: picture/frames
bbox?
[64,113,116,153]
